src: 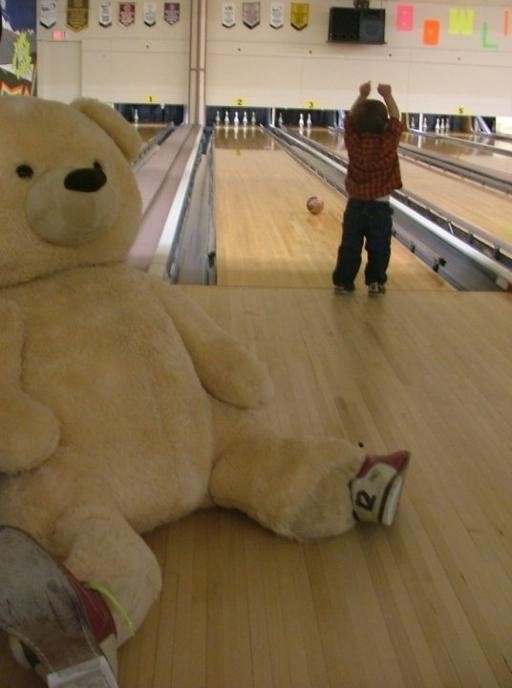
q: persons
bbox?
[331,80,404,298]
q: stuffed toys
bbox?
[0,94,414,688]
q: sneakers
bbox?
[334,284,355,295]
[368,282,386,298]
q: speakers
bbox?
[359,9,385,42]
[329,7,359,41]
